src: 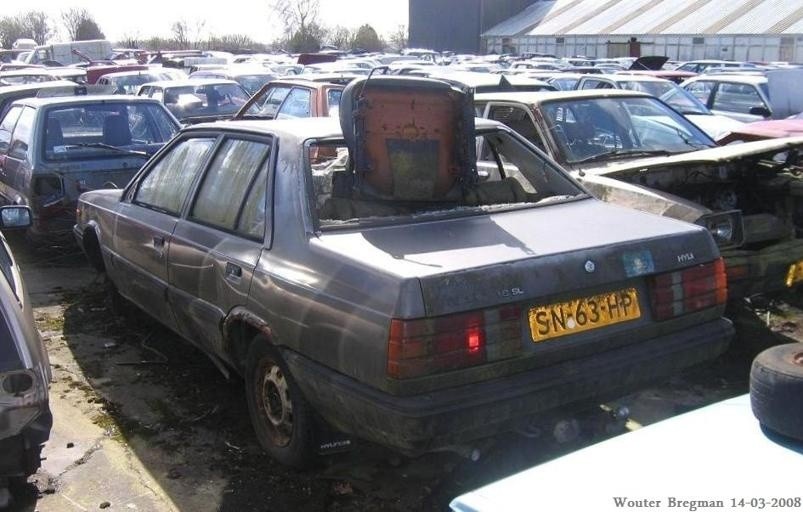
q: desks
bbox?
[450,390,803,512]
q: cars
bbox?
[1,224,55,481]
[0,36,801,307]
[73,110,738,470]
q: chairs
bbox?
[567,121,606,157]
[45,119,63,152]
[101,116,132,148]
